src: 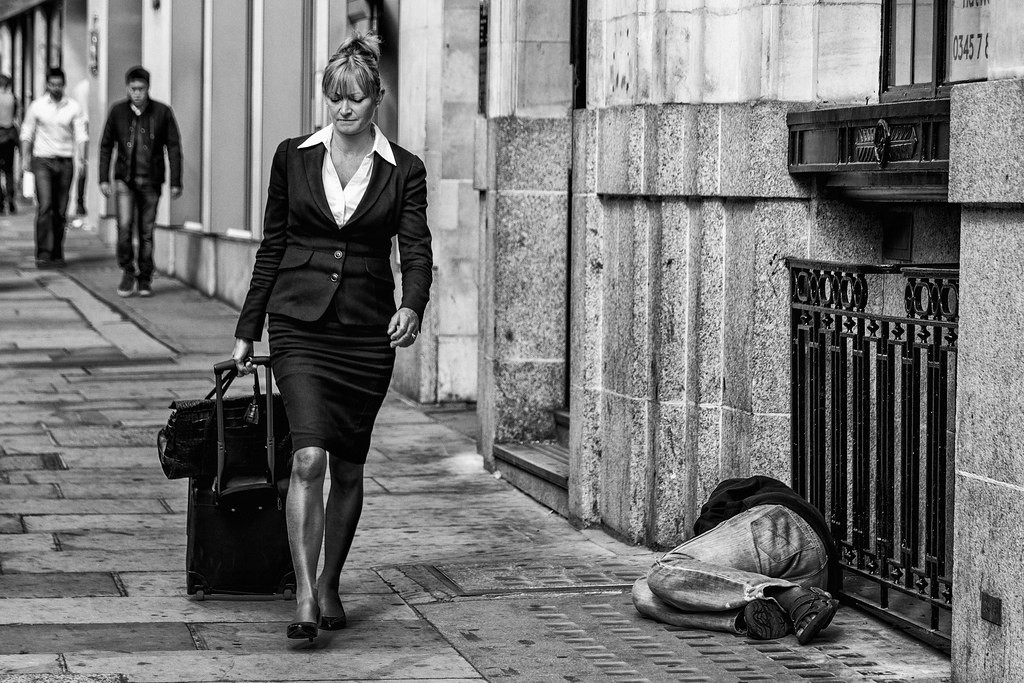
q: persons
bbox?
[0,70,23,156]
[72,79,87,228]
[630,474,840,646]
[227,29,433,644]
[19,67,91,269]
[0,64,17,214]
[98,65,184,298]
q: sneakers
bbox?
[117,271,135,297]
[137,279,153,297]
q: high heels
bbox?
[287,605,321,643]
[317,594,347,630]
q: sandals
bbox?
[743,599,792,639]
[789,586,839,646]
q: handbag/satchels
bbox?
[23,171,36,198]
[156,364,293,479]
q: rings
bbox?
[412,333,416,340]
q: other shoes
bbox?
[55,259,66,271]
[36,253,50,267]
[0,204,5,213]
[9,202,15,212]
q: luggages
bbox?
[186,357,298,602]
